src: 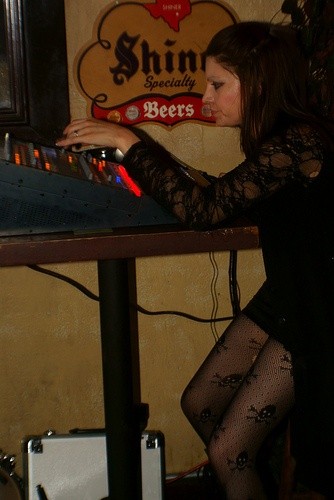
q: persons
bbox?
[56,20,334,500]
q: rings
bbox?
[72,130,80,137]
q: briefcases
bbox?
[0,428,166,500]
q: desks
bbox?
[0,211,261,500]
